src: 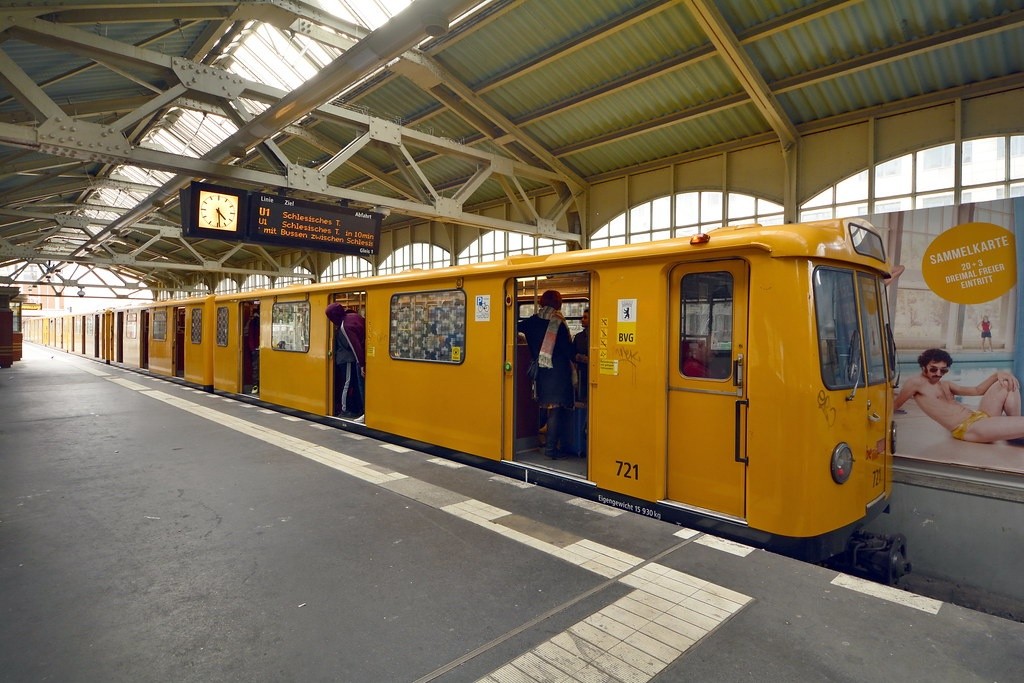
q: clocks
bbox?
[200,194,237,228]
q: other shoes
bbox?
[342,412,355,417]
[252,385,258,394]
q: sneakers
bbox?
[354,414,365,423]
[544,449,569,460]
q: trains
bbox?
[23,215,915,583]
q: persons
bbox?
[244,304,260,395]
[683,334,718,378]
[894,349,1024,443]
[325,303,365,423]
[517,290,571,459]
[572,308,591,454]
[976,315,994,352]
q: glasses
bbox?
[927,364,950,373]
